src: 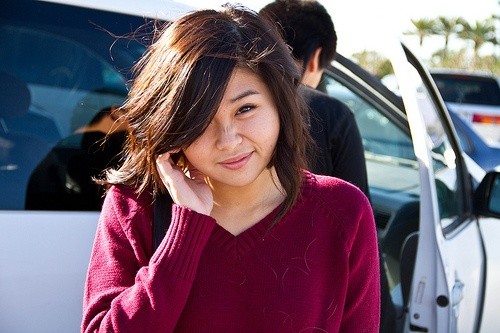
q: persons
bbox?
[81,3,382,333]
[23,105,136,211]
[252,1,398,333]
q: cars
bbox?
[0,1,499,333]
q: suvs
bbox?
[380,67,500,154]
